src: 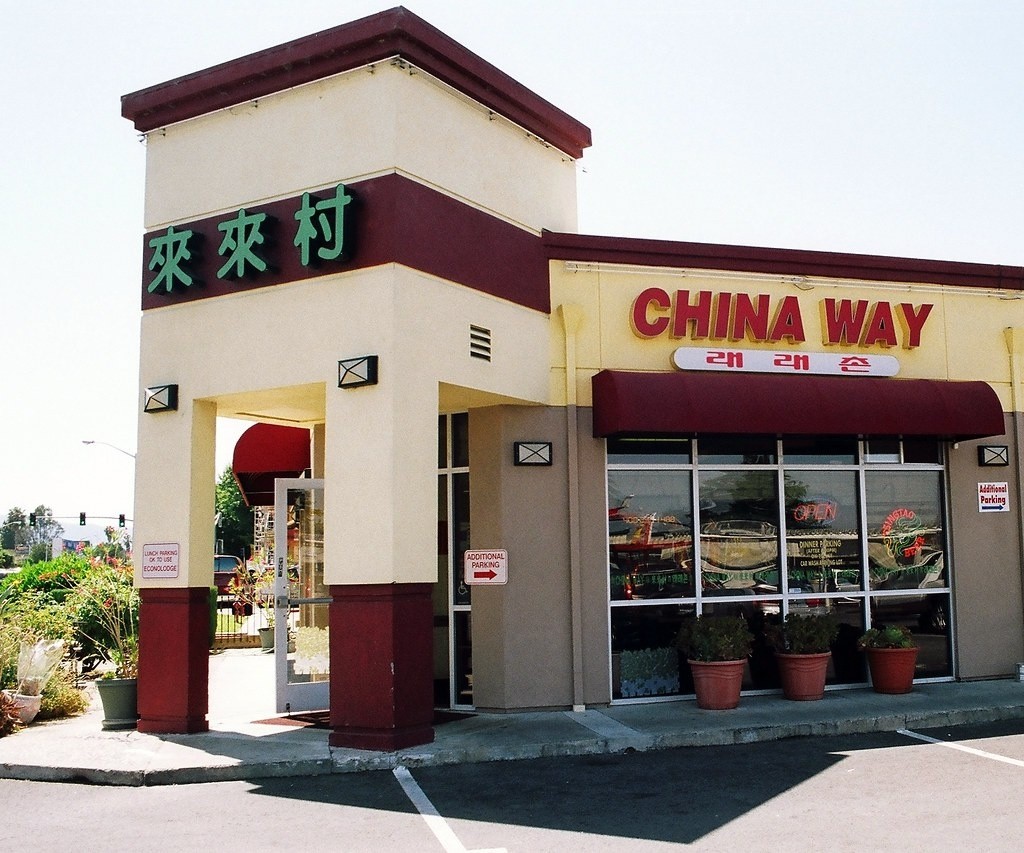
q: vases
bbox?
[94,677,138,731]
[3,689,42,726]
[257,627,290,653]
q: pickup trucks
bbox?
[215,554,247,596]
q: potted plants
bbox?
[765,611,840,701]
[859,622,923,694]
[677,614,757,710]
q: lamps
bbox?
[336,355,379,390]
[514,442,553,466]
[977,445,1010,467]
[144,385,178,413]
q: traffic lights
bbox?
[79,512,85,525]
[119,514,125,527]
[30,513,36,525]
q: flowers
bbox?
[39,536,138,680]
[223,541,299,628]
